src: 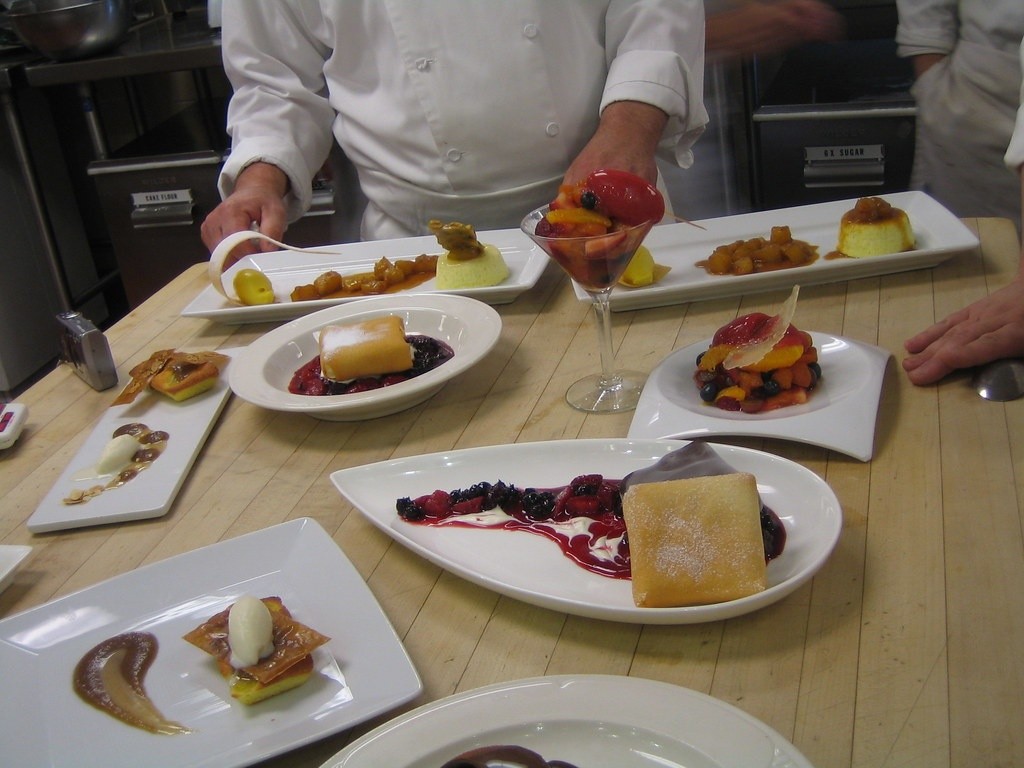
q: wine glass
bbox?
[519,202,655,416]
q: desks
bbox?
[0,13,156,309]
[0,218,1023,767]
[24,6,221,158]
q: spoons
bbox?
[972,358,1024,403]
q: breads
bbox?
[317,315,414,380]
[622,470,768,607]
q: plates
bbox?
[570,191,981,312]
[0,516,423,768]
[328,439,843,626]
[0,544,35,592]
[27,344,249,534]
[181,225,553,327]
[317,671,813,768]
[627,327,893,464]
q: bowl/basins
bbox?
[228,291,504,423]
[0,0,129,63]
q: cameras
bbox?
[56,310,119,393]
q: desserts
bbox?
[107,347,230,406]
[837,196,914,256]
[428,220,509,290]
[182,595,332,705]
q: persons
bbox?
[200,0,711,269]
[705,0,1024,386]
